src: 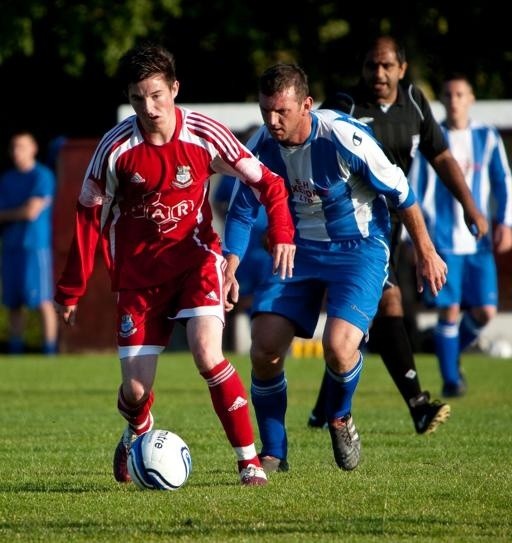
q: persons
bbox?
[409,77,511,396]
[54,46,296,489]
[221,63,448,475]
[308,39,488,434]
[0,133,58,354]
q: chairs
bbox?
[411,401,451,435]
[328,413,360,470]
[112,410,154,482]
[258,455,289,475]
[443,375,467,396]
[240,464,267,485]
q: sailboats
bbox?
[127,429,192,492]
